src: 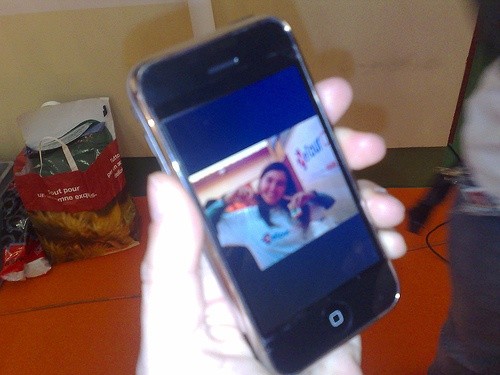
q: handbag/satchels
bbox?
[11,100,142,265]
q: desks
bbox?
[0,146,469,375]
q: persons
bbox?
[130,56,500,375]
[203,166,341,277]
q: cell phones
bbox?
[126,14,402,375]
